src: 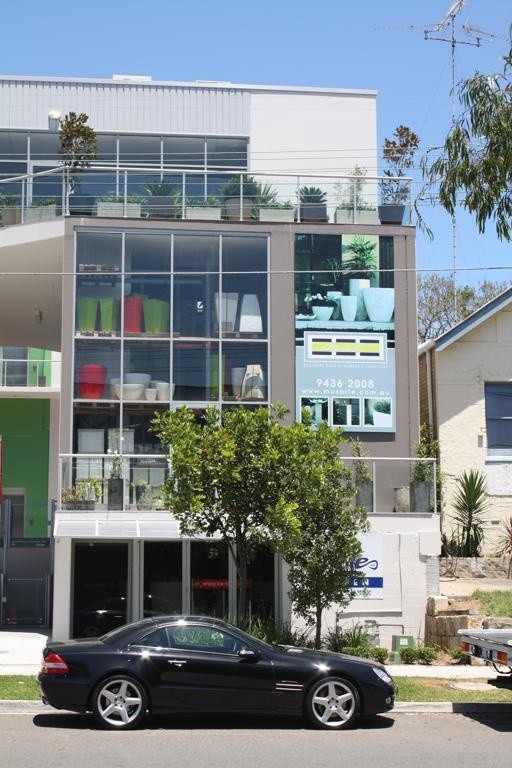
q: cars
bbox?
[35,614,399,730]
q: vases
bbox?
[362,287,395,322]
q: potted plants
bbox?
[332,124,421,228]
[1,111,99,225]
[96,175,329,222]
[62,427,153,510]
[347,420,444,513]
[310,234,378,321]
